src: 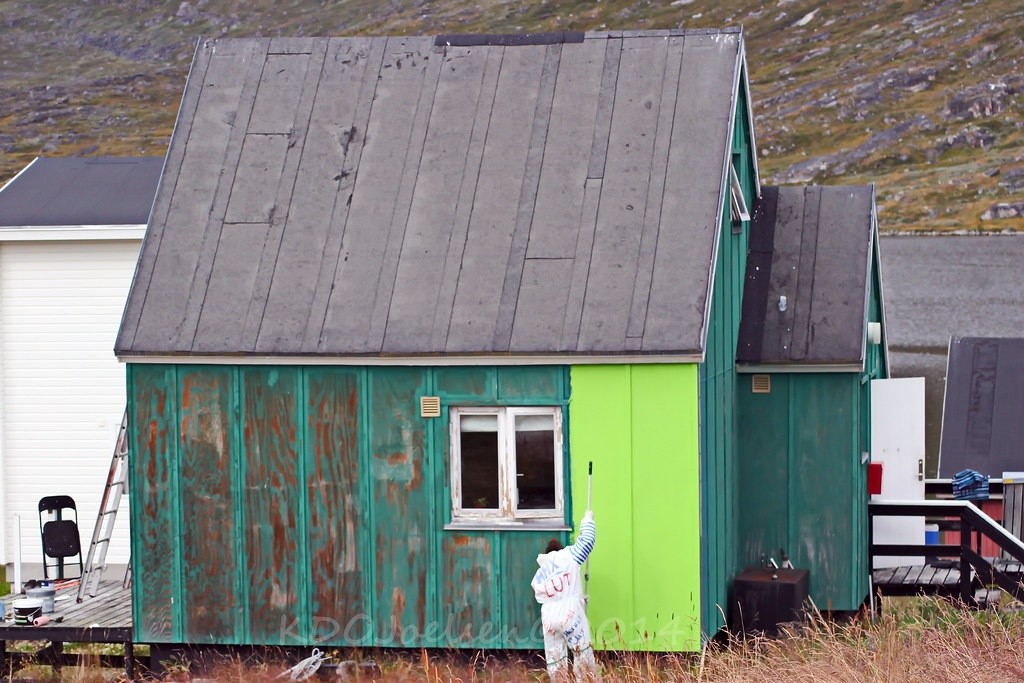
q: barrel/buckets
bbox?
[12,598,43,625]
[25,589,56,613]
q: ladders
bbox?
[76,403,131,603]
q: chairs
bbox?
[38,495,85,580]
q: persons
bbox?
[530,508,599,683]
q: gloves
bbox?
[585,508,594,519]
[584,594,589,603]
